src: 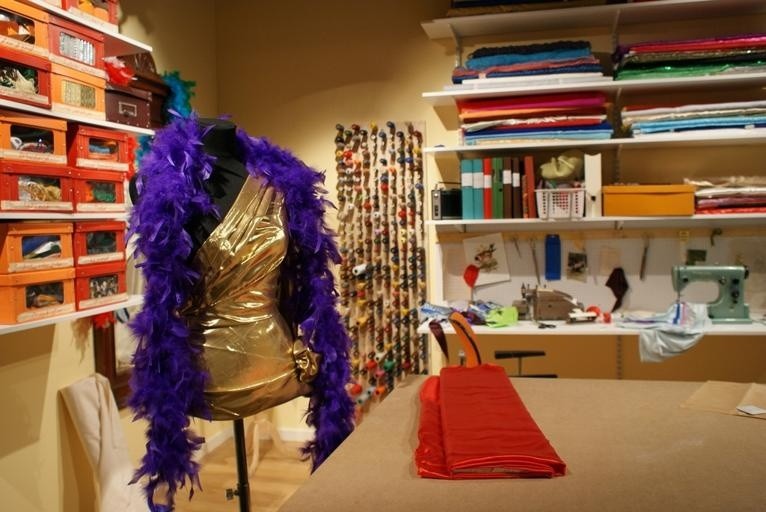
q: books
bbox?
[459,154,536,218]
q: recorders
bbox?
[432,182,462,220]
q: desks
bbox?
[266,364,766,511]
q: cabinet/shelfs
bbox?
[414,1,766,346]
[0,1,155,338]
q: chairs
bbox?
[448,306,560,379]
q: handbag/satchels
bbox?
[416,310,567,481]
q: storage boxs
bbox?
[35,0,63,10]
[70,221,128,265]
[68,120,130,173]
[75,263,128,310]
[0,270,75,327]
[1,104,72,168]
[103,83,155,129]
[1,158,75,212]
[0,44,51,110]
[600,181,699,220]
[63,2,120,33]
[68,164,126,213]
[47,12,110,78]
[0,0,51,59]
[1,220,75,273]
[51,58,109,124]
